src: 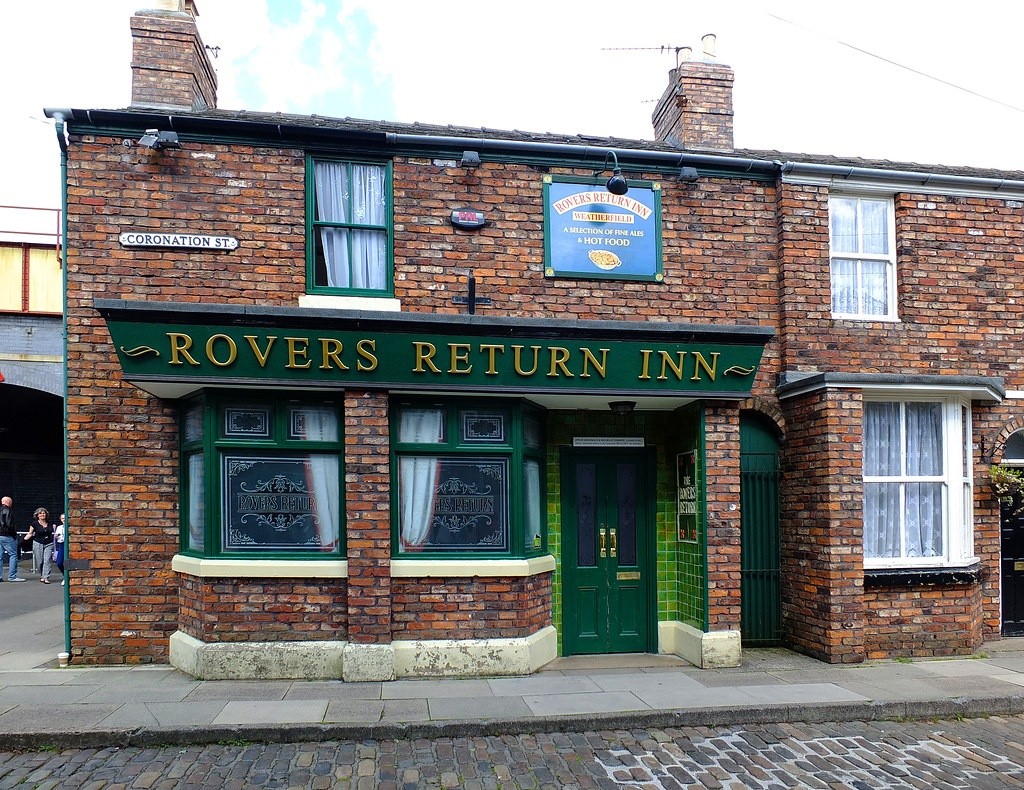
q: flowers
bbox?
[986,465,1024,506]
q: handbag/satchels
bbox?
[52,551,58,563]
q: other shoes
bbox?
[61,579,65,586]
[40,575,44,581]
[8,578,26,581]
[43,580,50,584]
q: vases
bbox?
[989,484,1019,496]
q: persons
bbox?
[21,507,54,584]
[53,513,65,586]
[0,496,26,582]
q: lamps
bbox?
[138,128,182,151]
[678,167,699,184]
[593,151,629,195]
[461,151,481,168]
